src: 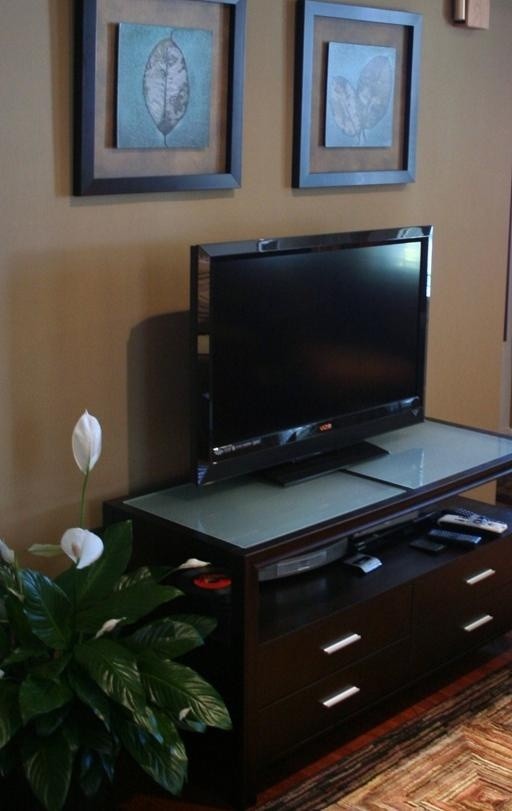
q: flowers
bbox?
[0,405,234,807]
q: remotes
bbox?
[438,514,508,535]
[455,507,489,520]
[427,527,481,547]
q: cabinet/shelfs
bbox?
[98,416,511,810]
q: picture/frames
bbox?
[290,1,424,190]
[71,0,248,196]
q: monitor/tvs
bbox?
[190,223,433,488]
